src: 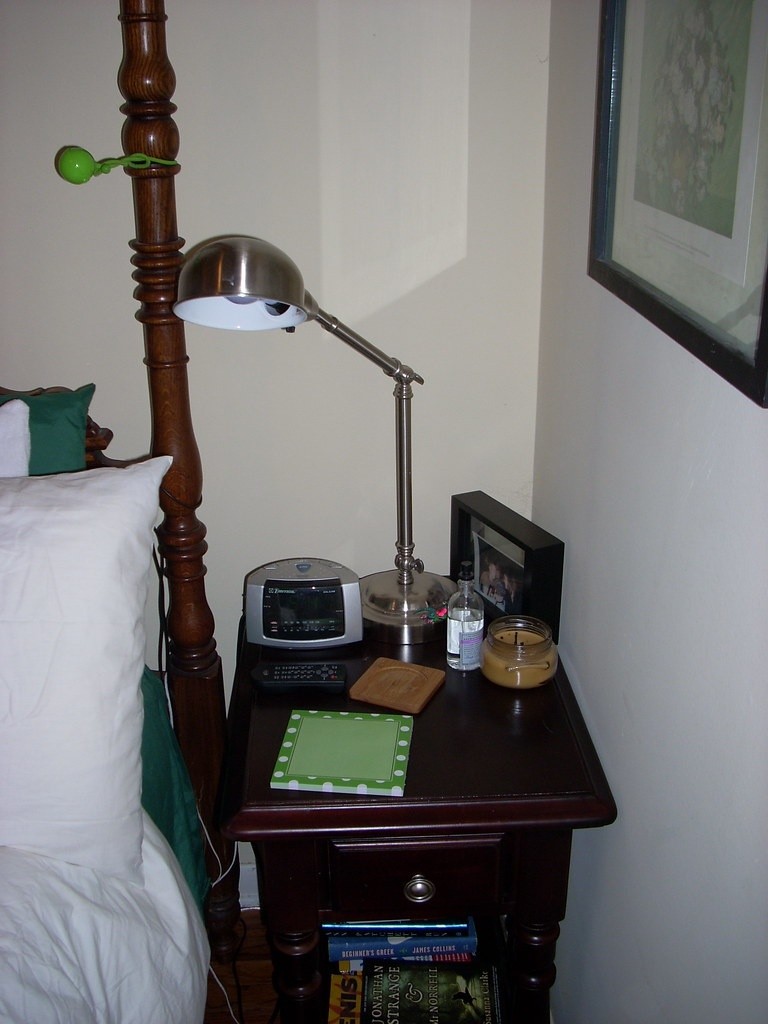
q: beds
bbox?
[0,386,211,1024]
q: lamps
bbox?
[174,237,459,628]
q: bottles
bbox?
[446,562,484,670]
[479,614,558,688]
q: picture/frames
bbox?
[584,0,768,409]
[450,490,565,644]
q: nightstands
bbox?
[218,624,618,1024]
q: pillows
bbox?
[0,382,96,477]
[0,456,172,886]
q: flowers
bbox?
[414,602,452,625]
